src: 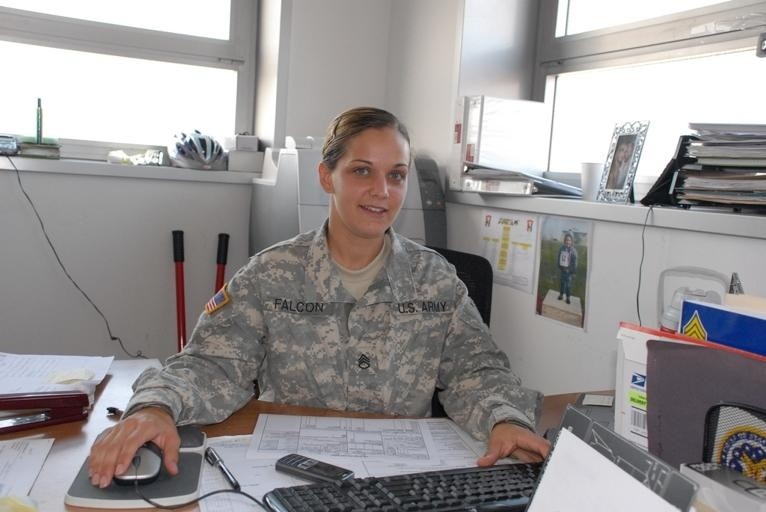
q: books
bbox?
[672,122,766,212]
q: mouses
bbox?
[114,441,162,486]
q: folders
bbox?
[461,161,584,198]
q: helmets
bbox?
[169,130,227,170]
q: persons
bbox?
[87,105,551,490]
[606,142,628,189]
[616,142,634,190]
[557,231,577,305]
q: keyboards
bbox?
[263,461,546,512]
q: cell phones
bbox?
[276,454,355,484]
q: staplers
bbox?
[0,390,89,434]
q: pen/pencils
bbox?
[205,447,239,489]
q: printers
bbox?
[248,136,447,255]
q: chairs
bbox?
[424,244,493,418]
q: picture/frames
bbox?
[595,120,650,205]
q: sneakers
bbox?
[558,296,570,304]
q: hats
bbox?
[564,229,574,247]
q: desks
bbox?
[0,358,615,512]
